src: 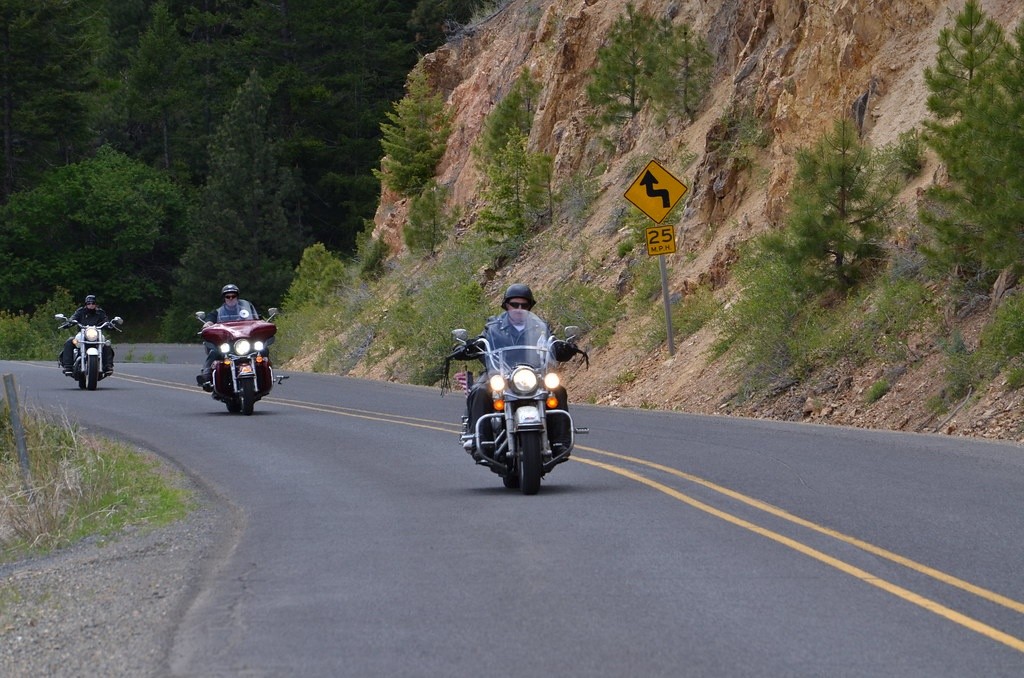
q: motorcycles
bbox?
[54,314,123,391]
[445,326,589,494]
[195,308,289,415]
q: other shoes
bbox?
[63,369,73,373]
[553,446,570,457]
[104,367,114,374]
[474,449,484,460]
[196,375,213,384]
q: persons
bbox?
[197,284,269,385]
[454,283,578,459]
[61,295,116,374]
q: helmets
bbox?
[501,284,536,312]
[222,284,240,294]
[85,295,96,302]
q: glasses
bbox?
[507,302,529,309]
[86,303,95,305]
[225,295,237,300]
